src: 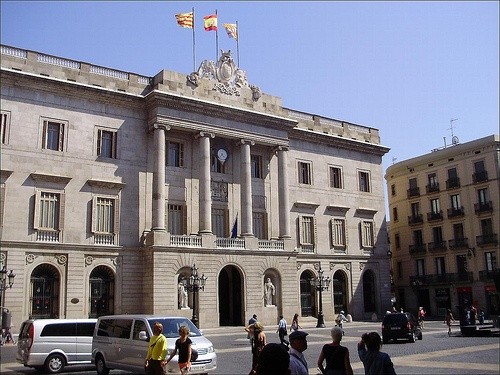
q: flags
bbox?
[203,14,218,32]
[174,11,193,30]
[221,23,237,41]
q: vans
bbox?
[91,314,218,375]
[16,320,99,373]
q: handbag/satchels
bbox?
[192,349,198,361]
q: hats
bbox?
[289,331,309,341]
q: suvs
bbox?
[382,312,422,343]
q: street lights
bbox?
[310,269,331,328]
[0,266,16,345]
[181,263,208,327]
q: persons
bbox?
[0,324,16,345]
[290,313,302,331]
[276,315,289,346]
[287,331,309,375]
[165,326,193,375]
[333,303,486,335]
[248,314,258,325]
[264,278,276,307]
[317,327,354,375]
[358,332,397,375]
[249,322,267,375]
[255,342,292,375]
[178,278,191,310]
[144,322,167,375]
[245,324,257,353]
